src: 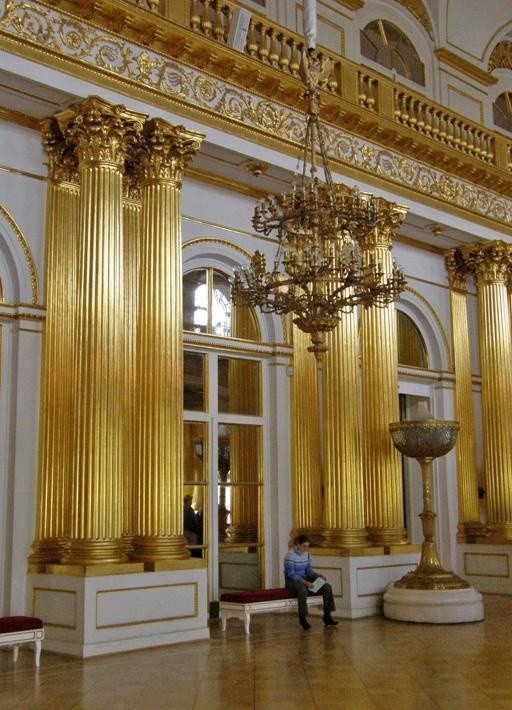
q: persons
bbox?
[183,494,200,555]
[218,503,230,543]
[284,535,339,630]
[196,506,203,520]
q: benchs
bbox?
[0,615,45,667]
[218,587,324,635]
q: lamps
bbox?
[387,400,459,457]
[226,0,410,365]
[195,424,231,480]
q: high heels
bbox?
[323,616,338,627]
[299,619,311,630]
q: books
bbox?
[307,577,324,593]
